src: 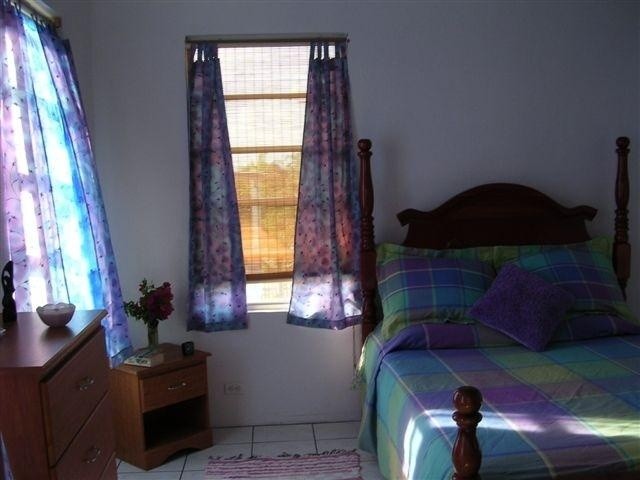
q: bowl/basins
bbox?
[36,301,76,329]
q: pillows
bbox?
[371,236,630,355]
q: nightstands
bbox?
[107,344,214,471]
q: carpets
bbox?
[202,448,360,480]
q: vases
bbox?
[147,320,159,349]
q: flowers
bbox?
[122,278,177,327]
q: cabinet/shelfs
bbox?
[0,311,121,478]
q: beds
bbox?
[355,133,638,479]
